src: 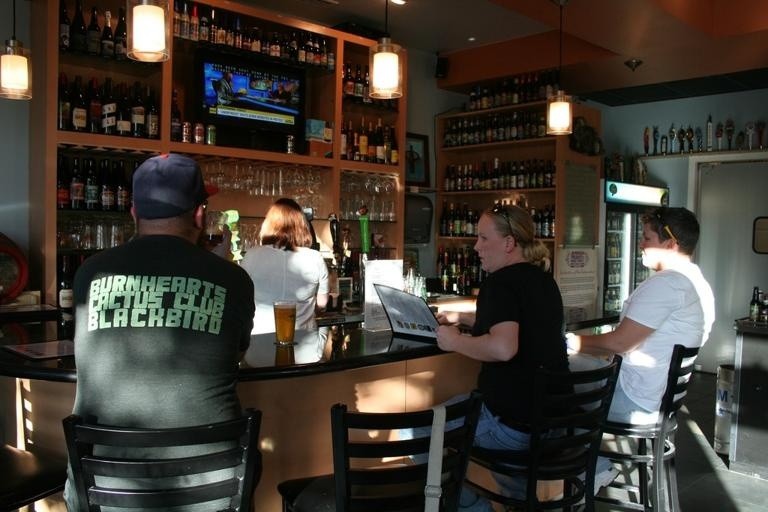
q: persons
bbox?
[239,197,331,335]
[268,80,299,105]
[405,145,421,174]
[61,153,263,511]
[216,71,241,108]
[539,206,716,506]
[398,203,576,512]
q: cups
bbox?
[272,299,297,345]
[273,343,297,368]
[328,292,339,308]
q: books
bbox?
[3,340,75,361]
[372,283,473,345]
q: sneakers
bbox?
[572,466,622,506]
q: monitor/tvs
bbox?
[192,40,307,138]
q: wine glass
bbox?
[191,155,398,264]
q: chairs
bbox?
[0,438,70,510]
[61,400,262,511]
[561,339,703,511]
[274,389,485,511]
[445,351,625,510]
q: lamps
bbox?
[365,0,405,102]
[0,0,34,104]
[122,1,176,66]
[542,0,577,136]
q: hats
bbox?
[132,153,219,221]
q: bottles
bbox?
[642,109,768,155]
[433,72,558,297]
[404,267,418,295]
[758,300,768,325]
[55,148,149,310]
[323,258,340,295]
[57,311,77,341]
[56,0,161,142]
[602,150,648,185]
[339,59,403,167]
[171,1,336,76]
[171,87,185,142]
[750,286,761,323]
[412,275,428,306]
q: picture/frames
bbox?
[406,132,431,190]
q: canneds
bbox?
[181,121,216,145]
[285,135,296,154]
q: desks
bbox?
[729,317,767,478]
[1,303,58,324]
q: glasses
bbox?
[492,203,518,248]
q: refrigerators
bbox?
[596,177,671,318]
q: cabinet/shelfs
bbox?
[431,96,605,306]
[28,1,412,345]
